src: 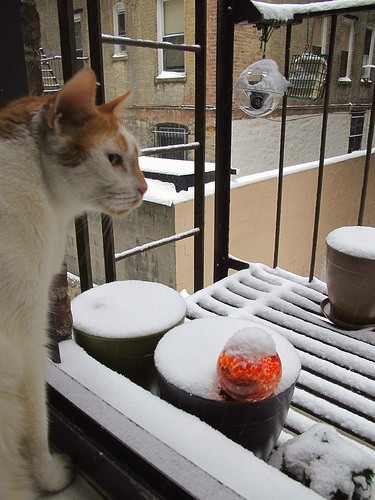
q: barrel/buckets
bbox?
[70,279,186,398]
[154,316,304,464]
[325,226,375,324]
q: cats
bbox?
[2,67,146,500]
